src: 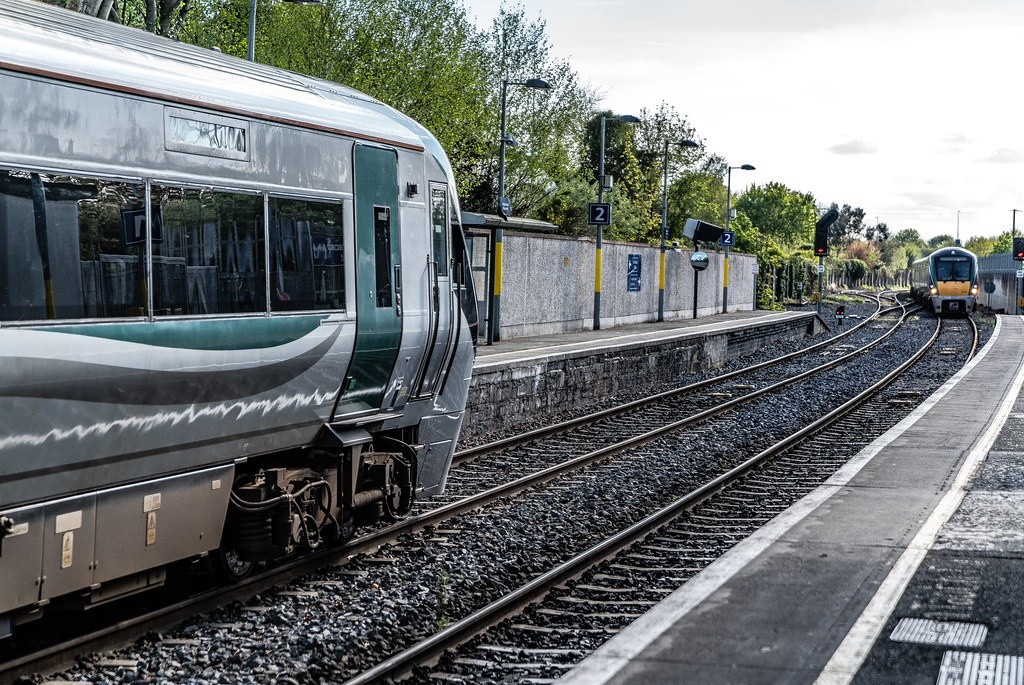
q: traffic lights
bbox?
[814,211,838,255]
[1012,237,1024,261]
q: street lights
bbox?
[722,164,756,313]
[587,115,643,329]
[659,140,699,320]
[493,79,555,342]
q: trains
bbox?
[909,246,980,315]
[1,0,482,648]
